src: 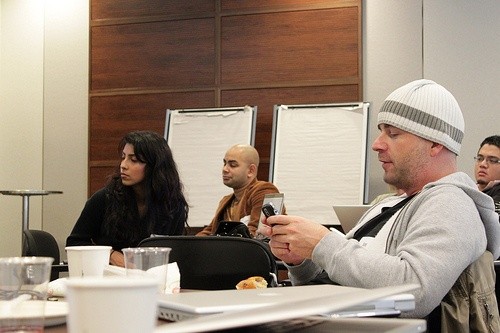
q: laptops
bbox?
[159,283,427,333]
[156,285,369,321]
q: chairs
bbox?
[22,228,59,282]
[137,236,279,290]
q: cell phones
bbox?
[262,202,280,226]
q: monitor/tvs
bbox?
[333,205,372,233]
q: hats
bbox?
[377,79,465,155]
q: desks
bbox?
[0,285,427,333]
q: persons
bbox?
[258,80,500,333]
[474,135,500,312]
[65,131,188,269]
[195,144,287,237]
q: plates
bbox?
[0,300,68,328]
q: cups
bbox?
[0,256,55,333]
[64,246,113,278]
[62,277,161,333]
[121,246,172,295]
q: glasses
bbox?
[474,156,500,164]
